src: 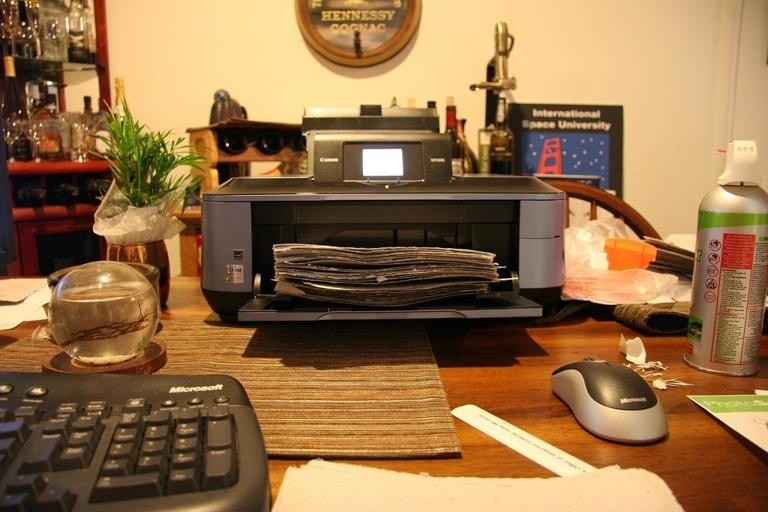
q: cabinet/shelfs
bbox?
[0,1,134,282]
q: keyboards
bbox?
[0,372,271,512]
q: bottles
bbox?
[113,77,135,162]
[685,184,767,373]
[491,97,515,172]
[0,1,94,161]
[444,96,481,177]
[426,101,437,118]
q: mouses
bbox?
[551,358,669,446]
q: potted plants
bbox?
[77,73,208,310]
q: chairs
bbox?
[538,176,666,245]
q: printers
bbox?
[199,130,567,322]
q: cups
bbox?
[45,262,164,339]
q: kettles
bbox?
[209,90,248,122]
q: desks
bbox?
[167,200,203,275]
[0,270,768,510]
